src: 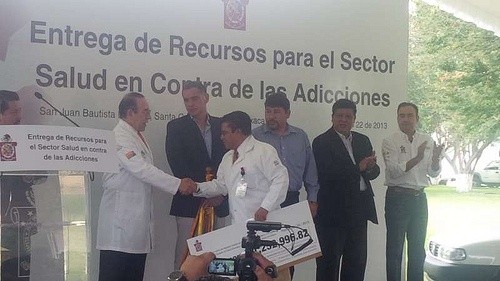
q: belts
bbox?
[388,187,425,197]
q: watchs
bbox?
[167,270,190,281]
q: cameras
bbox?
[208,257,260,281]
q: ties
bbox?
[233,151,238,162]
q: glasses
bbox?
[135,110,151,116]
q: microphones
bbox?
[34,92,80,128]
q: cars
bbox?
[472,159,495,186]
[423,218,495,280]
[438,173,471,191]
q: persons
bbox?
[0,89,41,281]
[311,99,380,281]
[250,94,319,281]
[182,110,290,281]
[95,92,191,281]
[164,82,232,280]
[382,102,444,280]
[168,244,293,280]
